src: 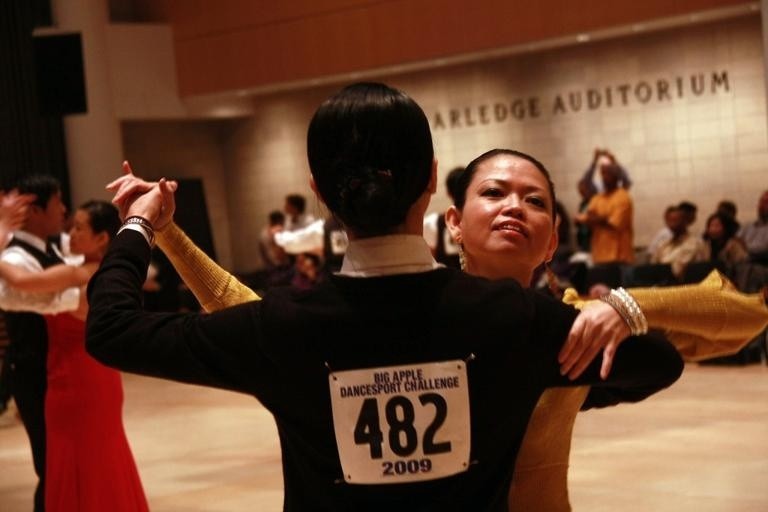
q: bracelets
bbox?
[598,286,649,337]
[116,214,158,250]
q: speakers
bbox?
[31,32,87,117]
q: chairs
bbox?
[559,260,765,367]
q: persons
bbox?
[255,143,768,320]
[0,186,37,254]
[80,78,686,510]
[102,145,768,511]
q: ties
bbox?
[43,241,69,266]
